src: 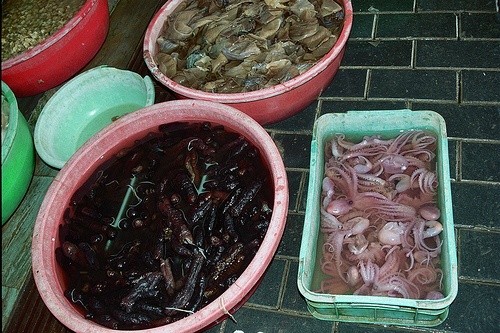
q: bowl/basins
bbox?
[143,0,352,125]
[0,0,109,98]
[2,80,36,227]
[32,101,288,332]
[32,65,154,169]
